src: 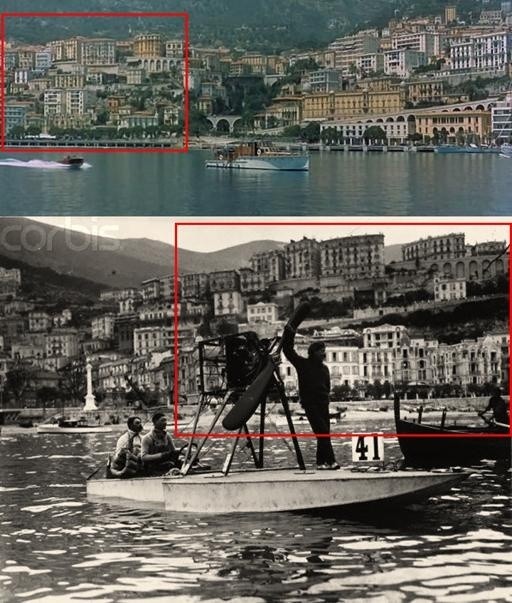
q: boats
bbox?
[78,467,482,516]
[292,406,348,419]
[57,154,84,167]
[499,142,512,157]
[202,136,312,172]
[386,388,512,473]
[33,421,113,435]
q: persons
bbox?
[116,416,144,458]
[478,387,509,429]
[141,413,176,466]
[282,324,341,470]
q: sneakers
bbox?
[317,461,340,470]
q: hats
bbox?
[308,342,325,354]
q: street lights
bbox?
[70,116,74,141]
